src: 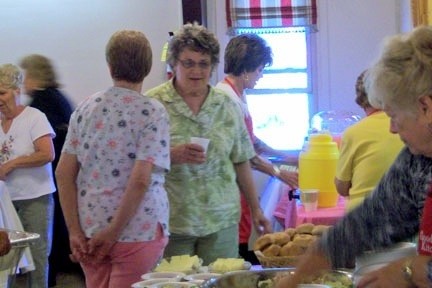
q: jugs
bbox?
[288,133,339,208]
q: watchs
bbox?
[403,255,418,288]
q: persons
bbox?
[214,31,304,267]
[1,227,12,257]
[144,23,273,270]
[334,67,408,224]
[53,28,172,287]
[0,63,59,287]
[265,27,432,288]
[19,53,74,286]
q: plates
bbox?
[8,232,40,247]
[131,256,251,288]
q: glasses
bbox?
[175,57,213,69]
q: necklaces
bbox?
[176,86,207,96]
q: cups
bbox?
[191,137,210,155]
[302,190,318,213]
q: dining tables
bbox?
[272,186,350,226]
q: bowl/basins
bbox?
[204,268,353,288]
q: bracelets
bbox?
[271,164,281,178]
